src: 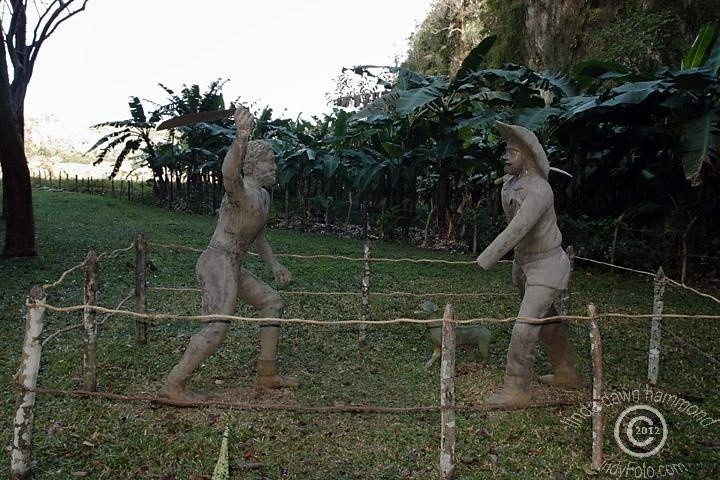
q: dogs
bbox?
[413,300,492,368]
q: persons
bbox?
[475,119,584,407]
[149,102,305,409]
